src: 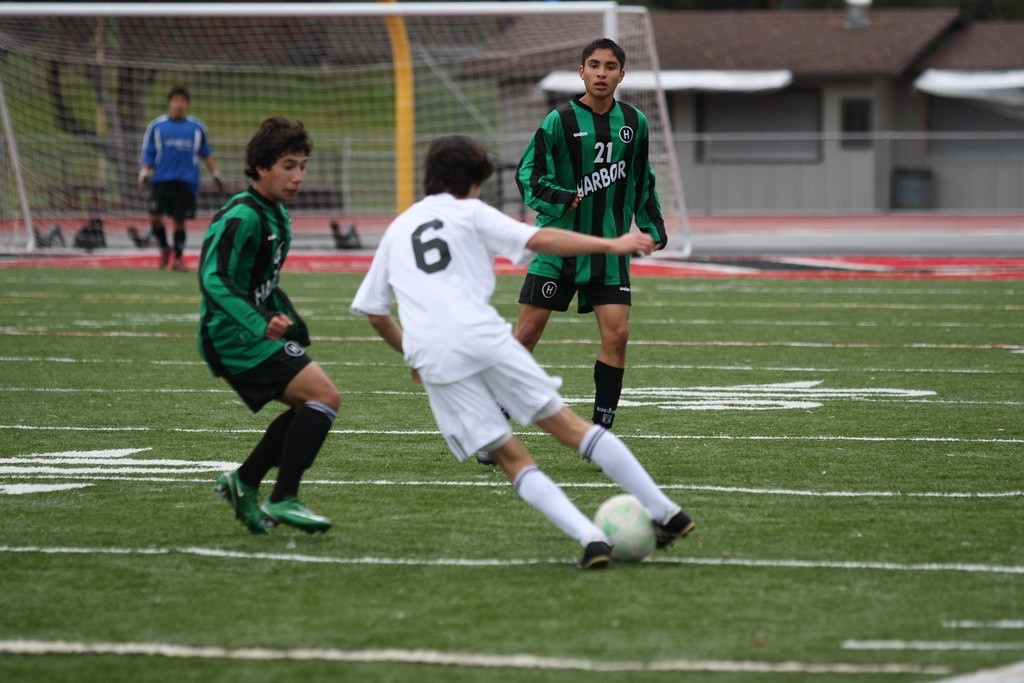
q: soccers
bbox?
[592,493,658,562]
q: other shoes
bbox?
[581,542,611,568]
[158,246,171,268]
[652,506,695,548]
[477,450,496,463]
[171,260,188,272]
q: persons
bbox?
[476,39,668,463]
[138,87,228,273]
[350,136,694,570]
[196,118,341,534]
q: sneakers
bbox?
[259,497,332,534]
[213,471,270,534]
